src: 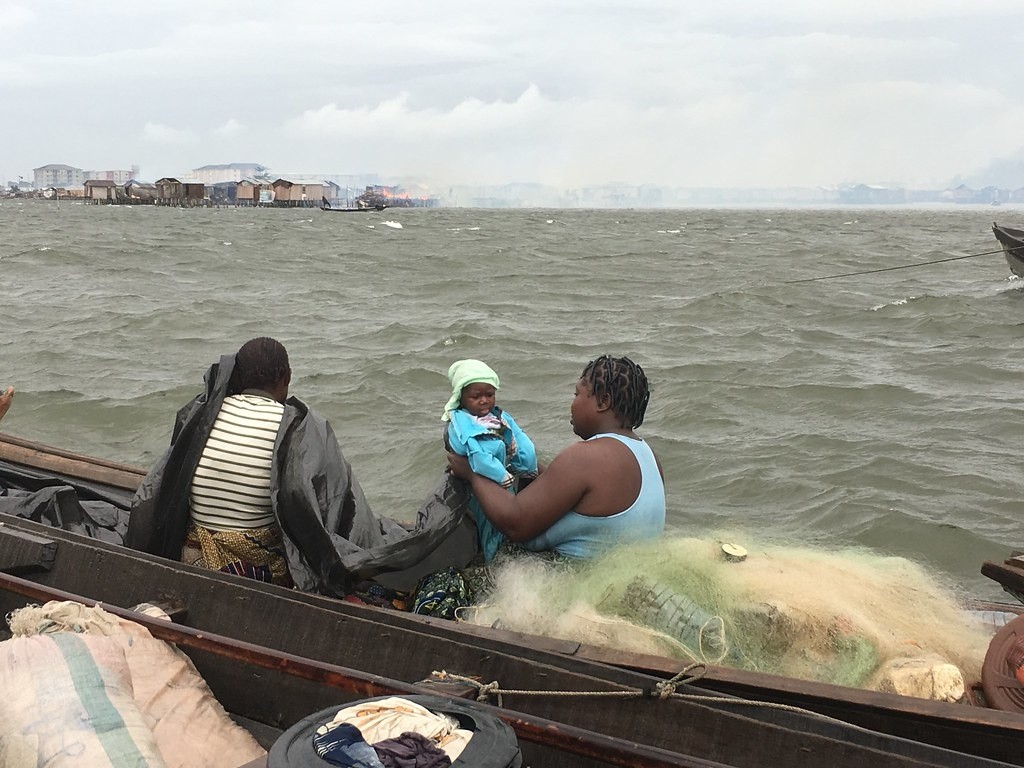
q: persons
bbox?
[322,195,331,209]
[180,338,292,581]
[440,358,541,560]
[446,354,666,571]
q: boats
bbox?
[986,223,1024,280]
[319,205,375,213]
[0,430,1022,768]
